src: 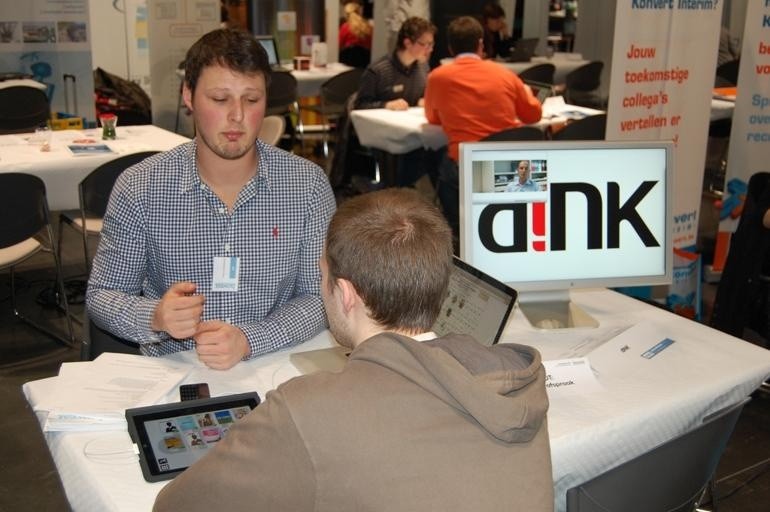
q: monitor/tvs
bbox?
[458,140,673,332]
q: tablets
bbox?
[124,391,260,483]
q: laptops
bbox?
[256,35,292,74]
[289,256,518,374]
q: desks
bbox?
[0,126,194,212]
[177,62,353,143]
[21,287,770,511]
[440,53,591,84]
[349,97,607,191]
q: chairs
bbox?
[267,72,307,157]
[80,318,144,361]
[55,151,167,328]
[565,61,604,107]
[566,396,752,512]
[0,173,76,368]
[432,127,543,242]
[0,85,50,135]
[549,115,605,140]
[302,70,363,159]
[519,64,556,98]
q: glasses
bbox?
[415,40,435,48]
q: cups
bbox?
[99,115,119,140]
[35,126,53,152]
[293,56,310,71]
[544,47,555,60]
[313,42,328,68]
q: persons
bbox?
[484,7,512,59]
[344,15,435,185]
[151,186,553,512]
[507,162,537,194]
[85,28,339,370]
[338,5,373,65]
[424,15,543,210]
[717,26,738,86]
